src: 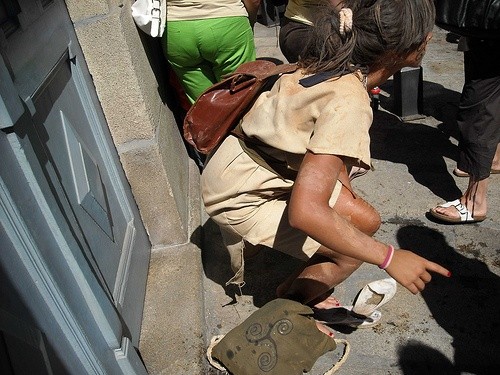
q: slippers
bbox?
[454,166,500,176]
[351,278,395,317]
[430,197,486,222]
[316,304,381,327]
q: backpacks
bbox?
[182,59,360,179]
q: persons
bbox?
[161,0,262,102]
[430,0,500,224]
[183,0,452,340]
[279,0,317,64]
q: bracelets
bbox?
[377,244,394,270]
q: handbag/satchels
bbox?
[211,298,337,375]
[132,0,167,37]
